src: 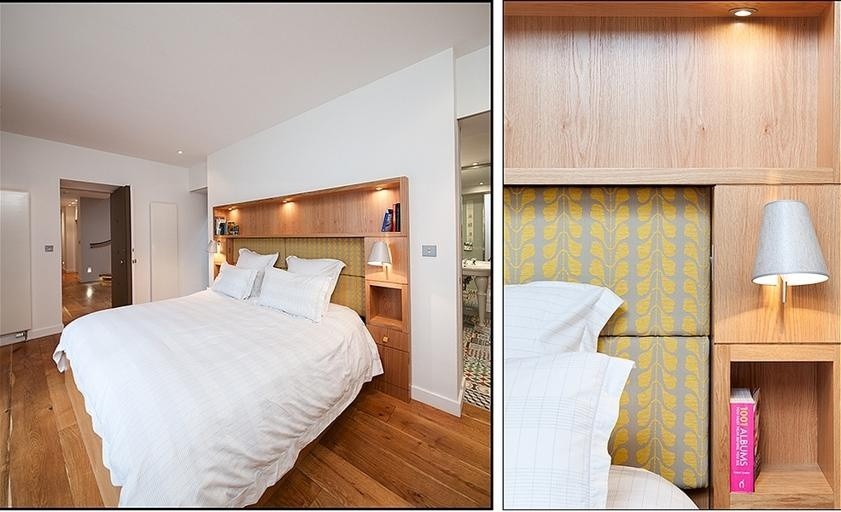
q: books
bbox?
[381,204,401,231]
[729,387,762,493]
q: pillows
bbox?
[503,280,625,351]
[503,352,635,510]
[208,247,347,323]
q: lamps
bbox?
[366,241,392,281]
[751,199,830,342]
[206,240,226,265]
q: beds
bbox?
[503,464,700,510]
[52,287,384,509]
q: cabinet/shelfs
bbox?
[212,176,411,404]
[503,0,841,509]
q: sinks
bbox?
[462,261,490,276]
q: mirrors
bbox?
[462,197,485,261]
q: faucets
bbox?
[471,258,477,265]
